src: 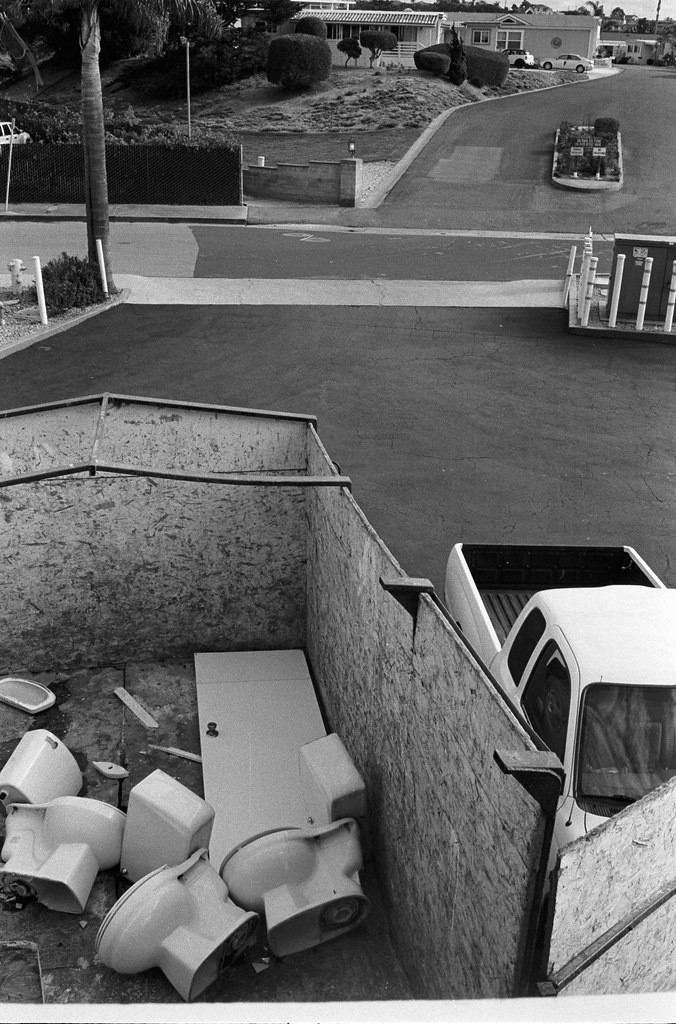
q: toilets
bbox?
[0,676,57,714]
[0,728,372,1003]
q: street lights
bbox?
[180,35,191,139]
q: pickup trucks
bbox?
[444,541,676,904]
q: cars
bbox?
[501,48,538,69]
[539,52,594,74]
[0,121,35,145]
